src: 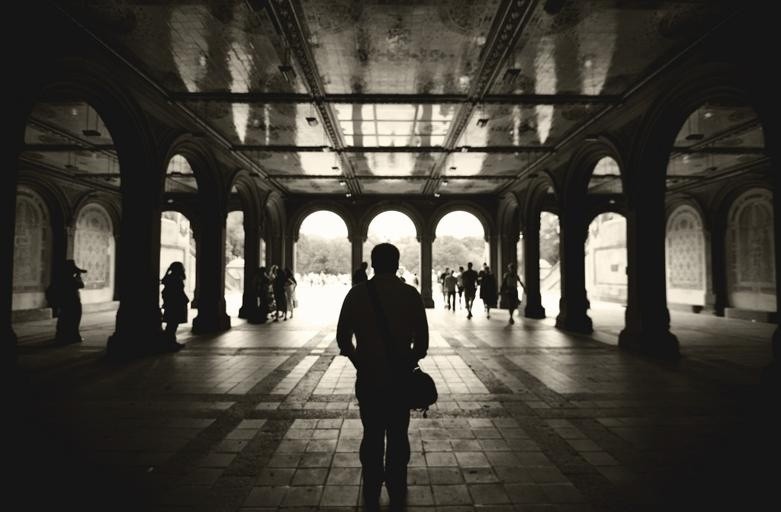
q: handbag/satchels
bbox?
[409,371,439,414]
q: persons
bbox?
[413,273,419,286]
[161,261,189,353]
[335,241,431,511]
[45,272,85,342]
[351,262,369,287]
[259,265,298,323]
[437,262,527,324]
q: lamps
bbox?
[701,141,718,175]
[63,138,79,171]
[685,109,703,140]
[278,37,353,197]
[434,50,523,198]
[81,103,102,136]
[104,155,118,182]
